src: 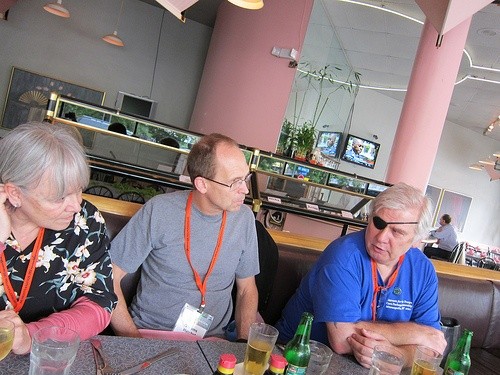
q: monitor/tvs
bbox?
[340,133,380,169]
[315,131,343,158]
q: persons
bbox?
[1,122,118,356]
[423,213,459,261]
[109,133,263,342]
[321,134,337,158]
[345,137,370,164]
[274,181,448,372]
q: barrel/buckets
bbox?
[438,316,462,366]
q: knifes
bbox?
[91,347,102,375]
[116,347,180,375]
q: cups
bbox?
[410,345,443,375]
[242,323,279,375]
[29,325,80,375]
[368,344,405,375]
[0,318,15,363]
[305,340,334,375]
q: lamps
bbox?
[43,0,71,18]
[227,0,264,10]
[102,0,125,47]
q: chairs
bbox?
[476,257,498,270]
[430,242,464,263]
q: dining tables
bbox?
[0,334,384,375]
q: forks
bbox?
[90,338,119,374]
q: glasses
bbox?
[203,172,253,191]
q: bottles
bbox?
[283,311,316,375]
[212,353,238,375]
[442,327,474,375]
[262,353,288,375]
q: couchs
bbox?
[100,211,500,375]
